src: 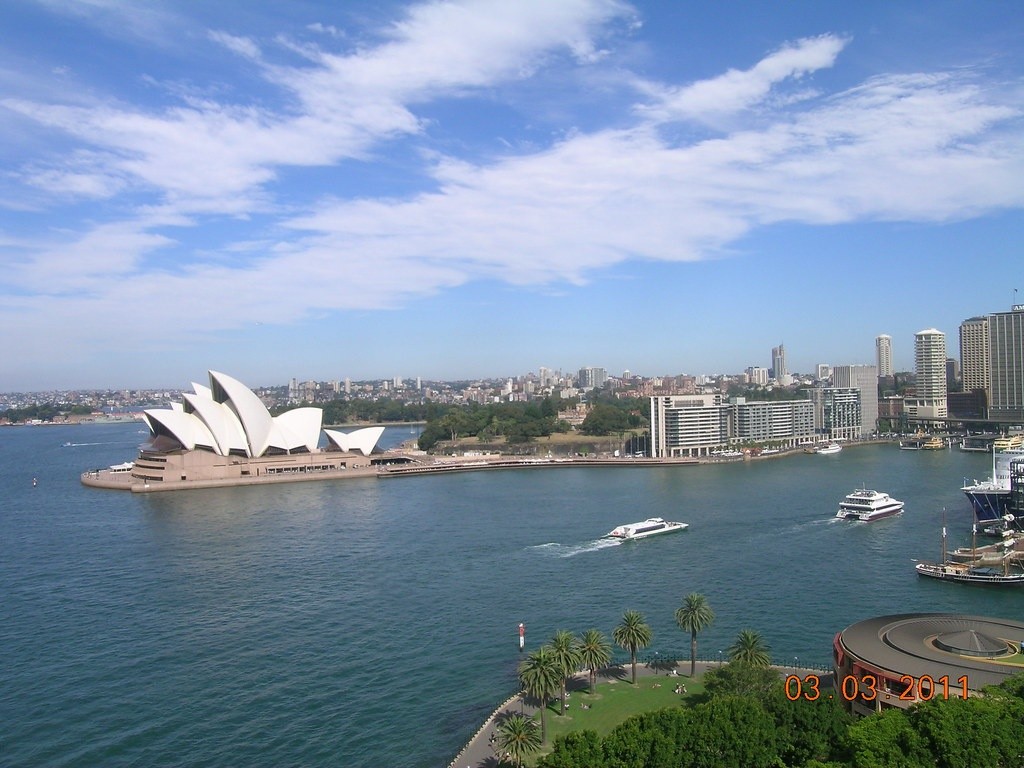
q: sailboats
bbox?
[915,500,1024,585]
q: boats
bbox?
[835,482,905,522]
[960,445,1024,536]
[922,437,945,450]
[817,443,842,454]
[61,441,76,447]
[600,517,689,542]
